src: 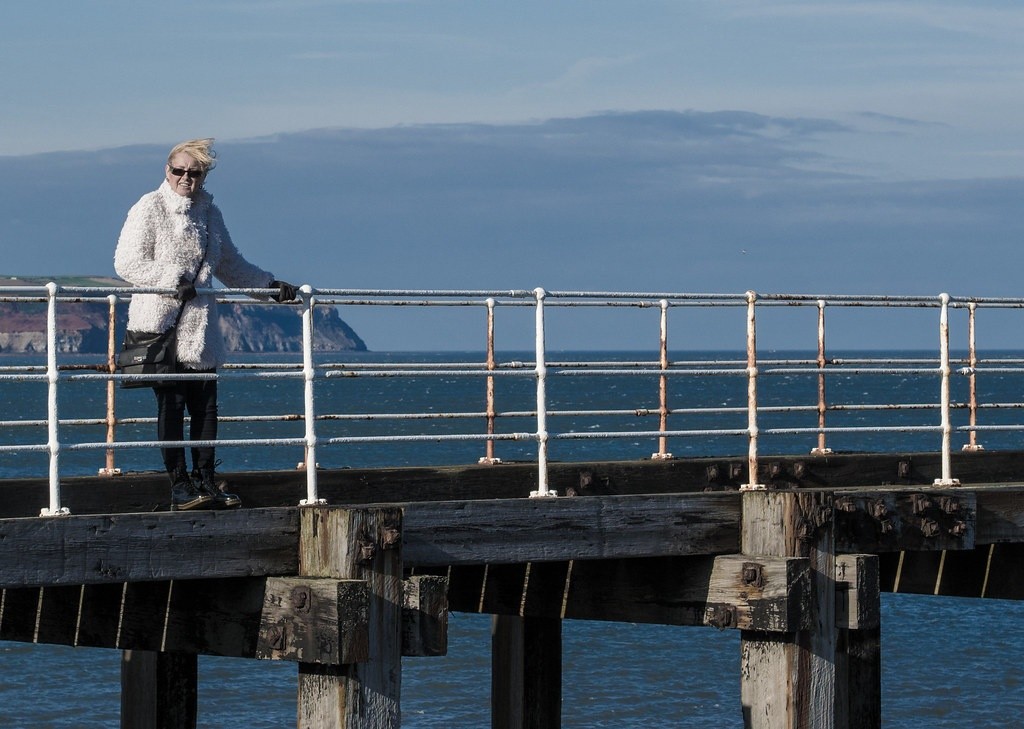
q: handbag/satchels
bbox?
[118,323,178,389]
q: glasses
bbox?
[167,163,205,178]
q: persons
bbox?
[113,134,303,512]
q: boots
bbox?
[164,462,241,511]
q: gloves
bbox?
[269,281,299,303]
[176,276,197,300]
